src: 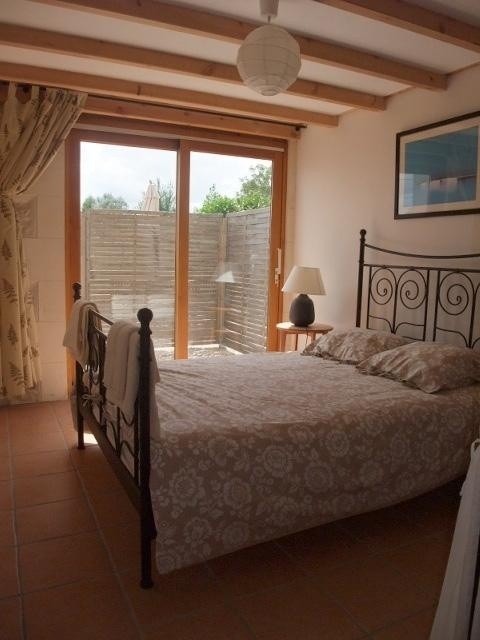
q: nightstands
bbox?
[276,321,333,352]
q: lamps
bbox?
[211,262,235,308]
[237,0,301,97]
[281,265,326,327]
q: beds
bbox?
[72,229,480,590]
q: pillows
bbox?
[355,342,480,393]
[300,326,404,364]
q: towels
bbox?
[102,319,161,441]
[62,300,103,372]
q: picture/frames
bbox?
[394,111,480,219]
